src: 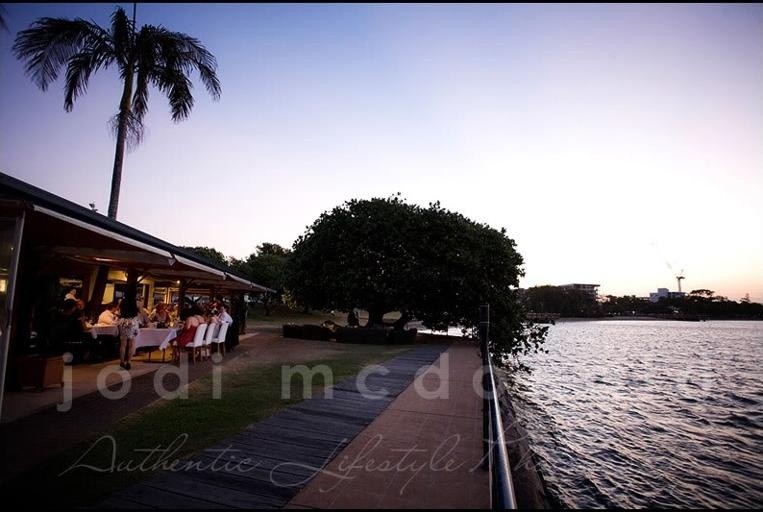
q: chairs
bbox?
[71,306,229,370]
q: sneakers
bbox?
[120,361,126,370]
[125,361,131,370]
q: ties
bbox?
[219,313,222,319]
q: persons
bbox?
[113,288,143,370]
[58,284,235,357]
